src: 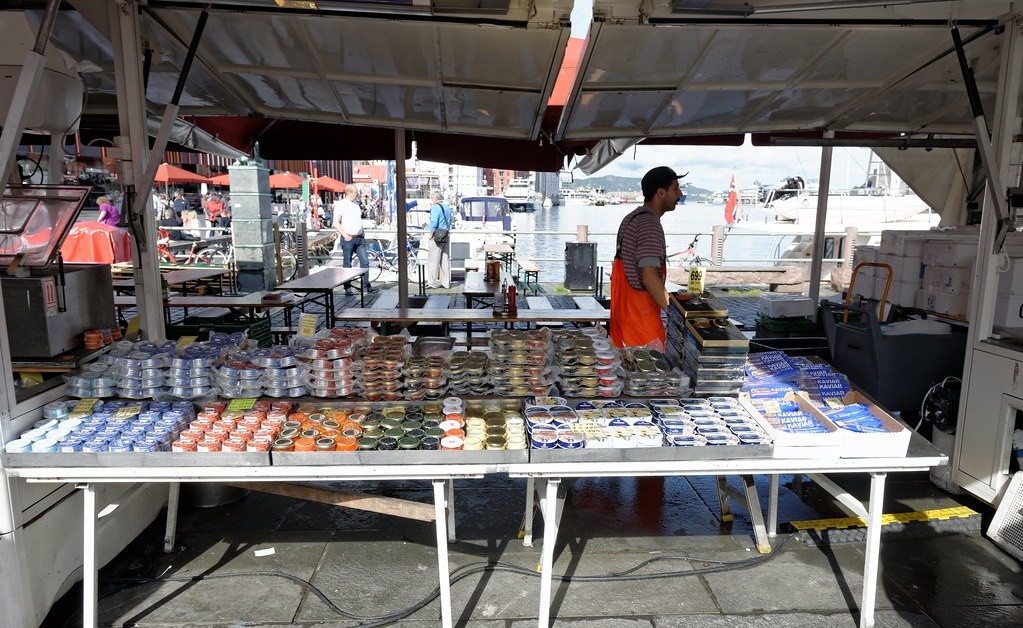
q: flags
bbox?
[725,175,736,225]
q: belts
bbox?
[353,235,364,238]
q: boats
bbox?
[386,172,446,229]
[724,150,940,281]
[453,175,516,248]
[502,176,543,211]
[678,191,688,204]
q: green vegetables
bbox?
[729,289,761,294]
[556,288,570,293]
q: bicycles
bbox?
[351,233,430,284]
[208,228,298,283]
[665,233,715,267]
[157,227,227,265]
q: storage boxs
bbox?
[686,318,750,353]
[874,277,922,306]
[880,229,963,258]
[851,272,876,299]
[852,246,881,274]
[923,234,979,266]
[760,295,816,319]
[923,265,972,294]
[669,290,730,322]
[738,391,844,459]
[877,254,926,283]
[165,316,272,348]
[918,289,968,316]
[796,390,912,458]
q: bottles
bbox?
[486,256,494,279]
[509,286,516,313]
[502,284,507,313]
[494,252,501,280]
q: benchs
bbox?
[356,291,399,332]
[271,326,299,345]
[237,296,304,320]
[571,296,610,329]
[515,258,540,296]
[525,296,562,330]
[191,290,269,324]
[417,296,452,337]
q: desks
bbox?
[4,243,948,628]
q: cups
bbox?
[494,293,504,313]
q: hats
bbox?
[641,166,689,190]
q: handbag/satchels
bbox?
[434,229,449,243]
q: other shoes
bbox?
[181,250,185,254]
[345,288,354,296]
[439,285,445,288]
[370,287,379,293]
[425,284,432,289]
[173,251,179,255]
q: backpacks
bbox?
[160,200,174,220]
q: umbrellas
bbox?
[270,171,315,208]
[208,174,229,185]
[314,176,347,203]
[154,163,213,200]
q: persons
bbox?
[152,190,201,254]
[96,197,120,227]
[195,187,230,235]
[333,184,380,295]
[425,193,451,288]
[610,166,689,351]
